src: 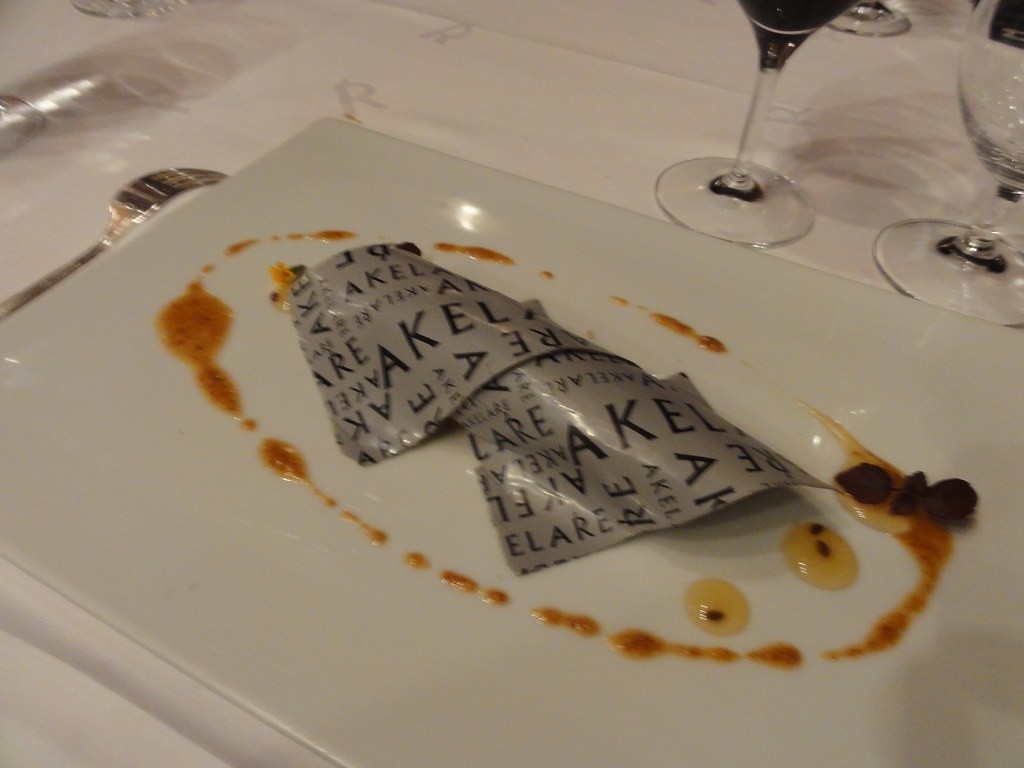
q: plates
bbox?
[0,118,1024,767]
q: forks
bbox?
[0,168,226,320]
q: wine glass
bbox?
[872,0,1024,328]
[656,0,860,249]
